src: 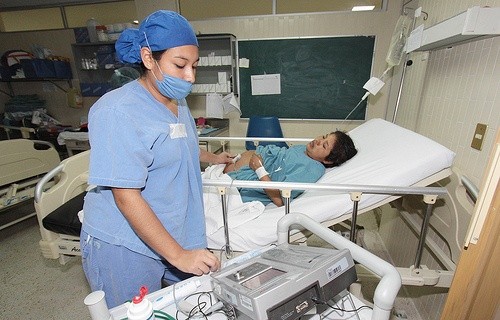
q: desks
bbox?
[84,212,402,320]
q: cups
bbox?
[83,290,110,320]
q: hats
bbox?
[115,10,199,63]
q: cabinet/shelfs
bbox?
[71,33,238,97]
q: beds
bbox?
[34,117,481,289]
[0,125,61,230]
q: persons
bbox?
[80,10,233,309]
[195,131,356,207]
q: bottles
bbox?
[87,18,98,42]
[80,53,98,69]
[97,25,109,42]
[126,286,155,320]
